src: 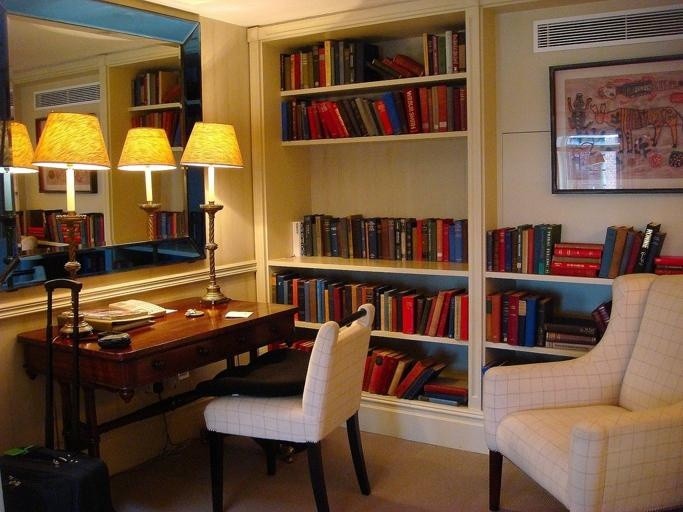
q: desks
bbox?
[18,295,299,465]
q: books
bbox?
[291,213,468,264]
[153,208,186,240]
[485,221,683,280]
[486,288,612,352]
[129,68,184,148]
[267,269,468,407]
[482,359,539,374]
[17,209,105,253]
[279,28,467,142]
[59,308,148,320]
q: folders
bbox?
[56,308,155,333]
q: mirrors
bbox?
[0,1,206,291]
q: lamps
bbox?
[0,120,39,258]
[117,127,177,239]
[31,113,112,338]
[180,122,244,304]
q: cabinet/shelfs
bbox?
[104,44,188,248]
[246,1,496,457]
[486,271,615,357]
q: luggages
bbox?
[0,444,117,512]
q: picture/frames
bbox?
[34,115,97,193]
[548,55,683,193]
[48,214,93,253]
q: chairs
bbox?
[193,303,374,511]
[483,272,683,512]
